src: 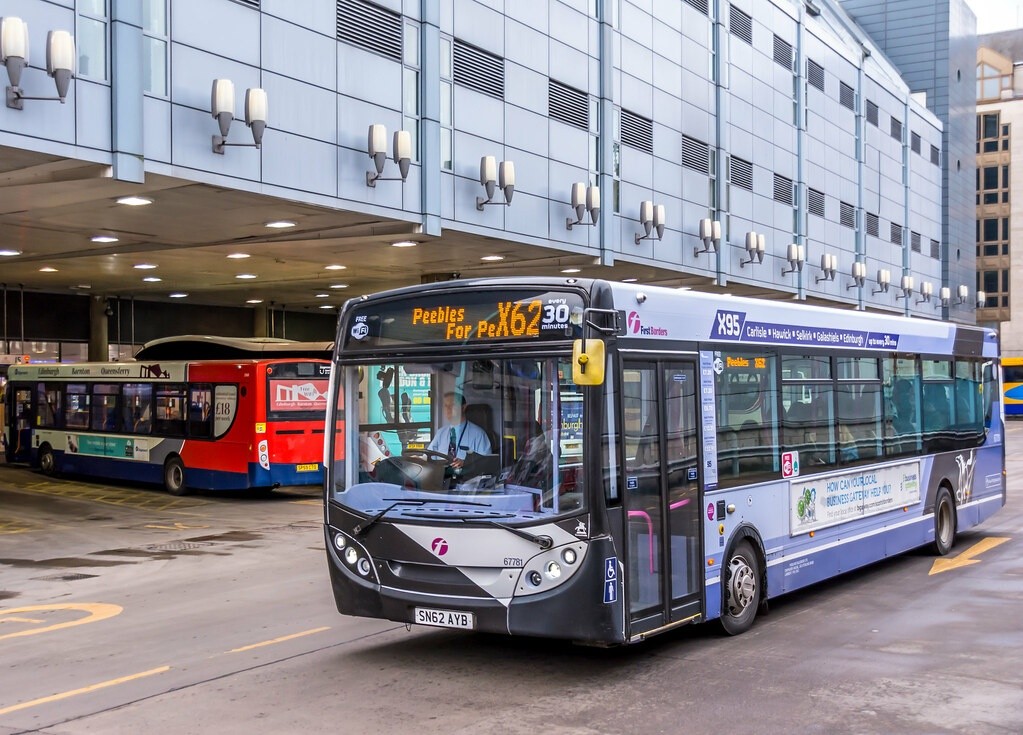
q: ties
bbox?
[447,428,456,461]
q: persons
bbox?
[805,394,944,464]
[411,392,493,469]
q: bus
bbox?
[323,276,1007,648]
[134,335,431,473]
[3,357,345,495]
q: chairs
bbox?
[465,404,499,454]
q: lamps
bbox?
[694,218,722,257]
[567,182,601,231]
[847,261,867,291]
[954,285,969,306]
[970,291,986,310]
[934,287,950,309]
[477,155,515,211]
[364,124,412,188]
[781,244,805,277]
[896,276,914,300]
[915,282,933,305]
[212,78,267,155]
[815,254,838,284]
[739,232,765,268]
[872,269,891,295]
[635,201,665,245]
[0,15,76,109]
